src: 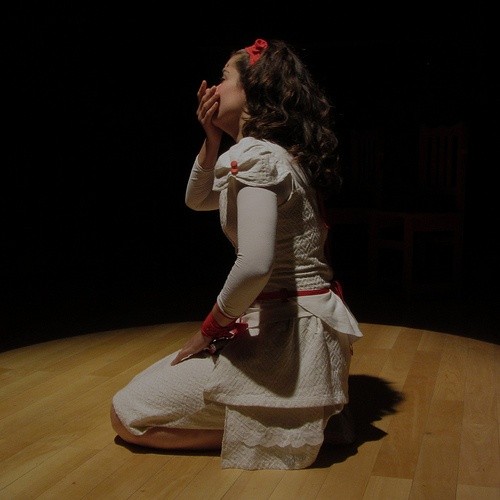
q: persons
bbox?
[111,38,364,469]
